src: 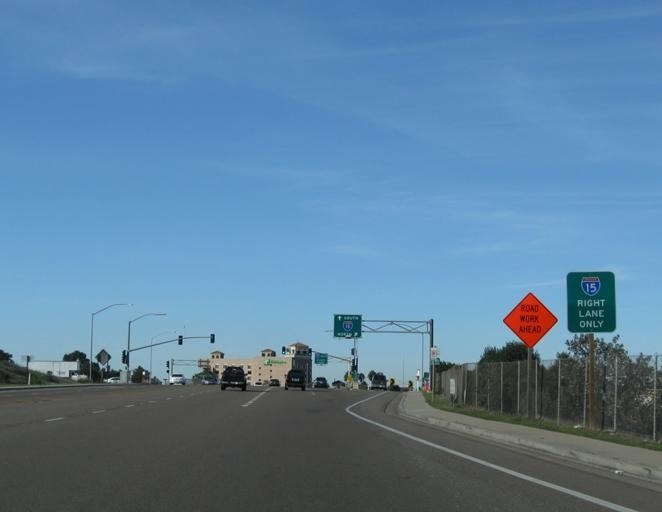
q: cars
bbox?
[285,368,306,391]
[169,372,185,385]
[369,374,387,389]
[202,375,217,384]
[269,379,280,386]
[221,366,247,391]
[314,377,328,388]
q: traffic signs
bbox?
[566,272,615,333]
[333,314,362,337]
[432,358,440,366]
[315,352,328,364]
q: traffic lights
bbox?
[211,334,214,343]
[122,350,126,363]
[179,336,182,345]
[166,361,169,367]
[352,359,355,367]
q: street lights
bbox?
[89,304,133,380]
[127,313,167,369]
